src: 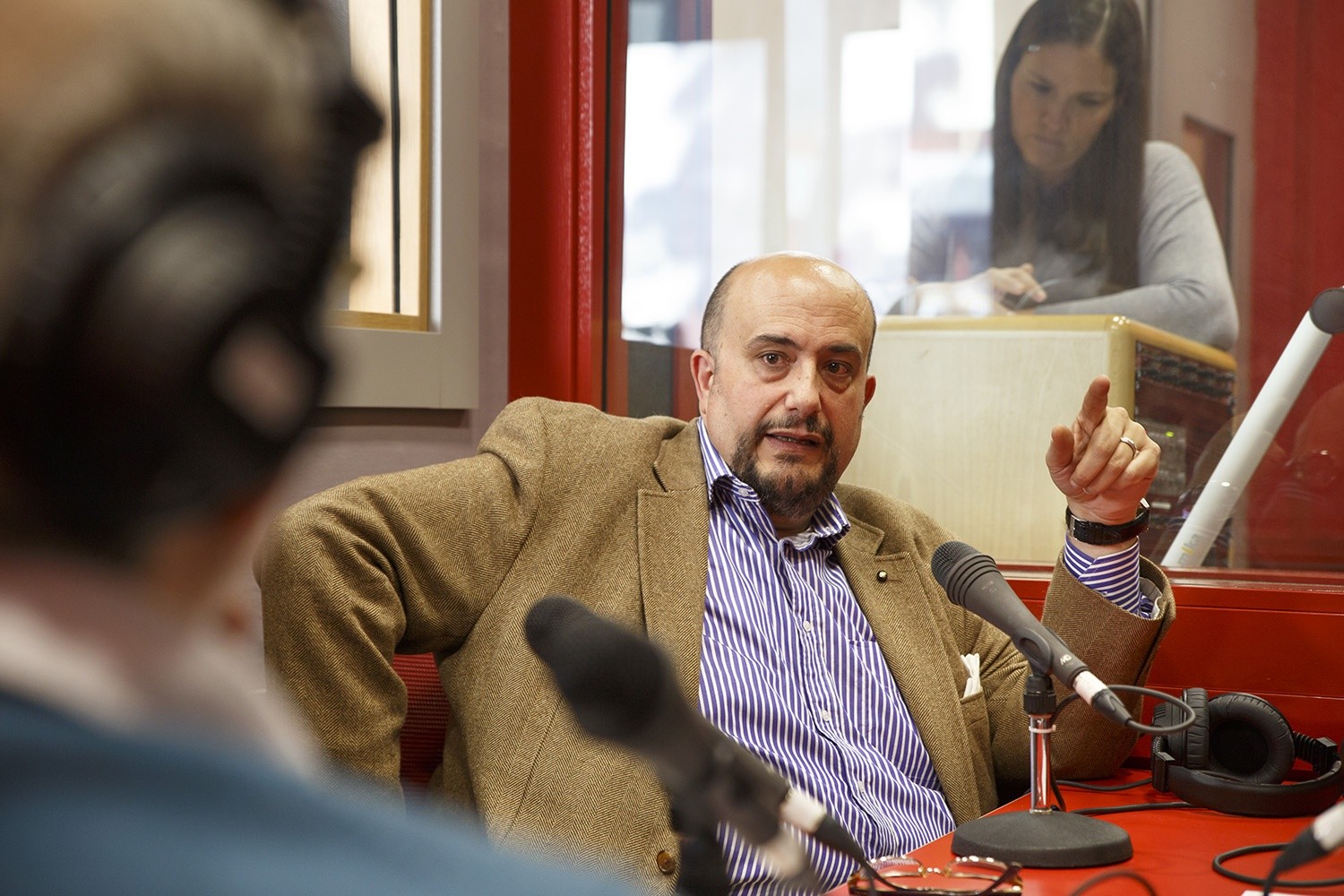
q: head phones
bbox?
[1,128,331,448]
[1151,689,1343,817]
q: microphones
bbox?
[523,596,863,860]
[931,541,1136,724]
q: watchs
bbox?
[1065,498,1151,546]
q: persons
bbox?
[0,0,645,896]
[255,254,1176,896]
[889,0,1241,350]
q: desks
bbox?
[821,759,1344,896]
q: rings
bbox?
[1120,438,1137,456]
